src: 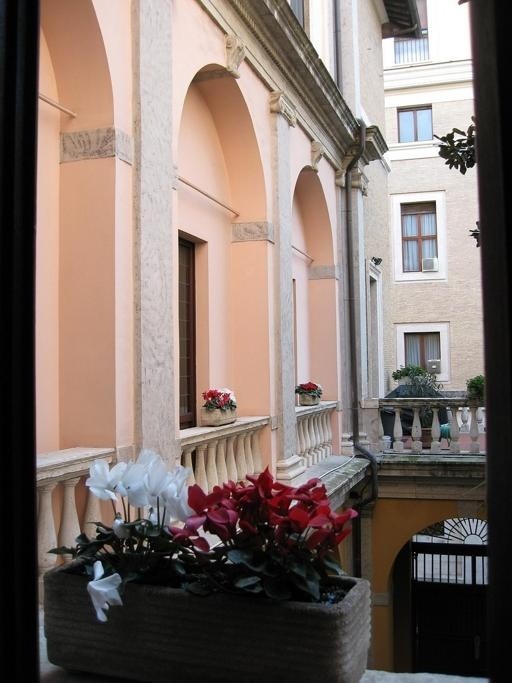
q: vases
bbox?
[300,393,320,406]
[201,407,238,427]
[42,550,376,683]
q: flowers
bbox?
[201,386,237,413]
[295,381,323,400]
[63,448,356,625]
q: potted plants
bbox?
[389,362,446,449]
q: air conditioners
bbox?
[421,258,439,273]
